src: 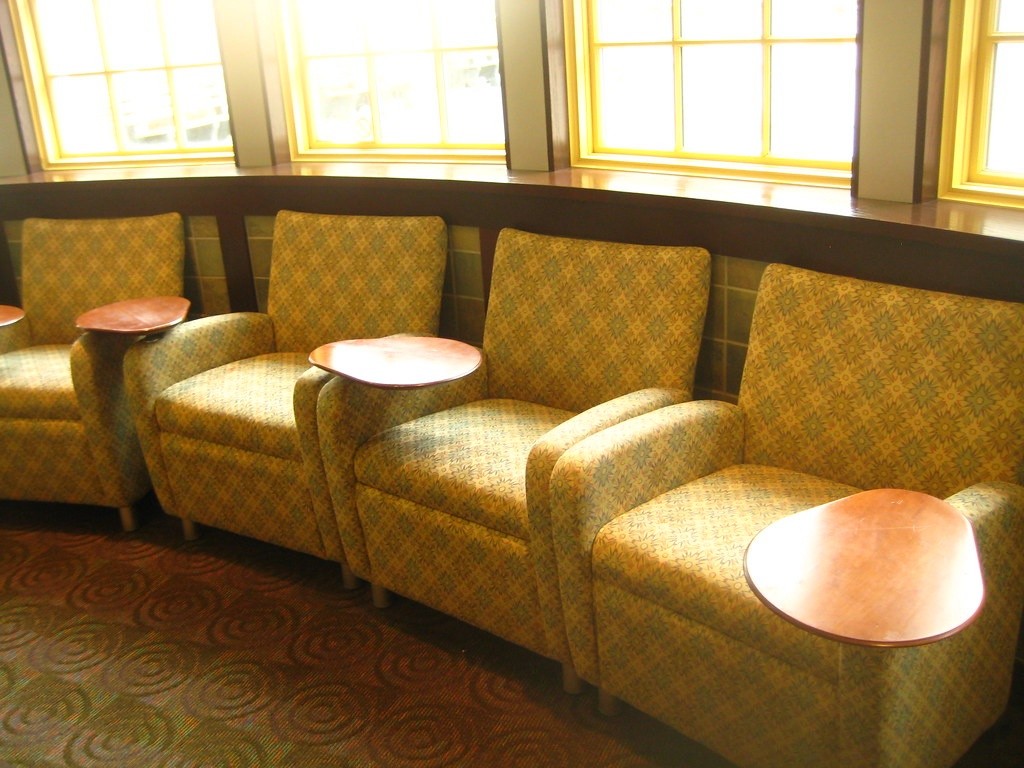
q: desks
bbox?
[0,304,23,326]
[308,336,482,389]
[75,295,192,343]
[742,487,987,647]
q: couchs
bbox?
[0,213,185,531]
[121,209,449,590]
[549,263,1024,768]
[316,228,711,694]
